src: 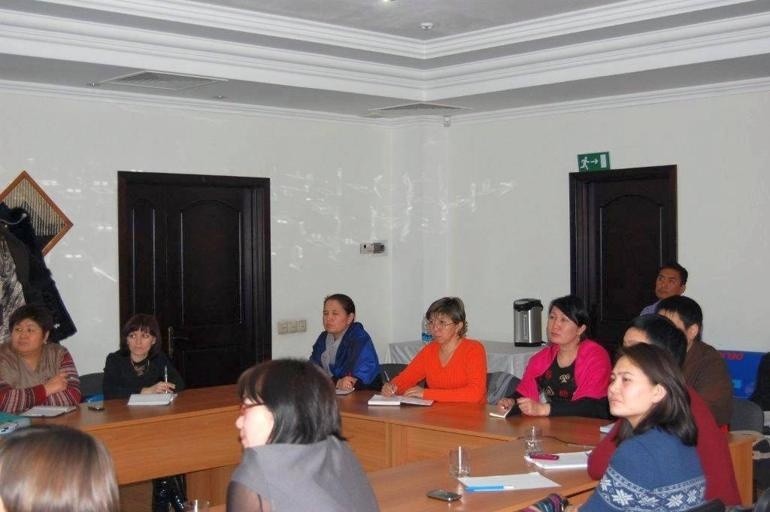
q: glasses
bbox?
[424,320,457,332]
[238,400,268,416]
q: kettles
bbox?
[513,298,543,346]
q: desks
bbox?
[337,387,757,511]
[0,383,245,512]
[363,438,603,512]
[388,338,549,381]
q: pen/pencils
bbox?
[382,368,396,400]
[465,484,516,492]
[163,365,169,395]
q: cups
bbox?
[448,447,472,480]
[525,425,543,454]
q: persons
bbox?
[0,304,81,413]
[226,359,380,512]
[382,297,487,404]
[654,295,732,432]
[587,313,743,508]
[0,425,120,512]
[102,313,199,512]
[641,262,688,315]
[498,294,612,420]
[310,294,383,392]
[565,343,706,512]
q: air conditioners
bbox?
[93,71,228,93]
[369,101,474,119]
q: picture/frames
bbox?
[2,170,73,257]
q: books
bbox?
[128,393,174,406]
[367,395,434,406]
[20,405,77,418]
[524,450,594,470]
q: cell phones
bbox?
[88,404,105,411]
[427,488,463,502]
